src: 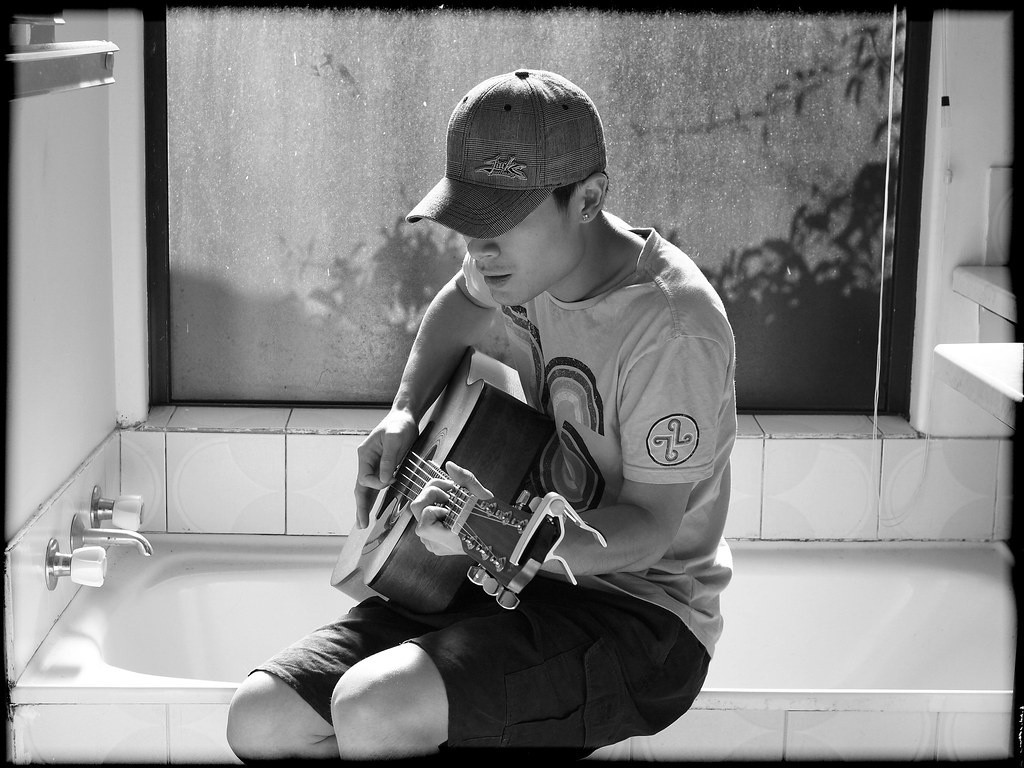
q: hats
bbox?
[406,69,606,239]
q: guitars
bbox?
[328,344,608,618]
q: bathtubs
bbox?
[12,538,1024,767]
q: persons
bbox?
[227,67,739,765]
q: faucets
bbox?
[69,512,155,558]
